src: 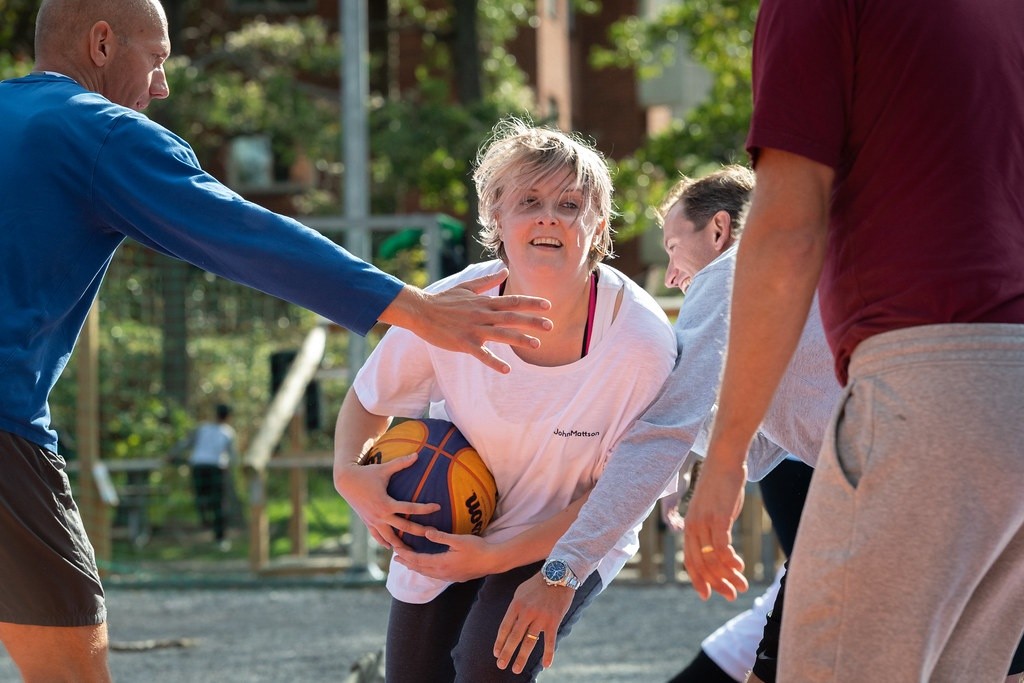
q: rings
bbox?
[526,633,539,640]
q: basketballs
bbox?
[366,419,497,553]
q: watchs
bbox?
[541,559,581,590]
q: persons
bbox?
[0,2,554,683]
[332,112,677,683]
[492,0,1024,683]
[160,405,235,553]
